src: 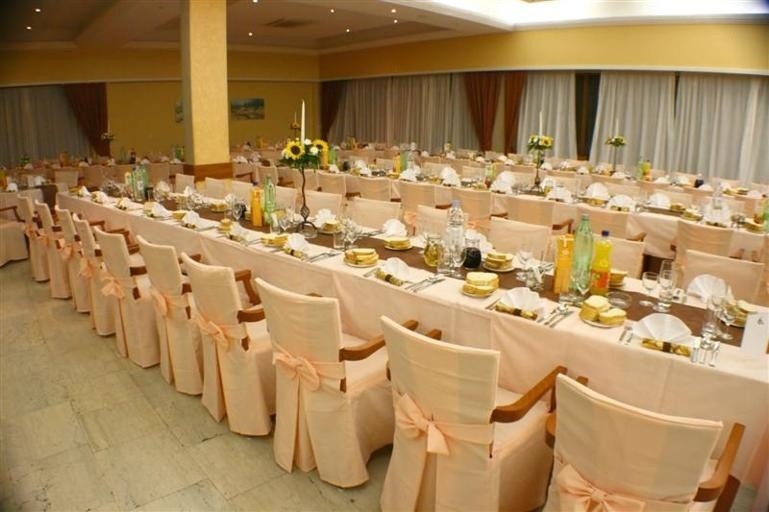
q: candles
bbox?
[538,111,543,136]
[301,99,305,140]
[614,117,619,136]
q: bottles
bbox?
[125,143,650,296]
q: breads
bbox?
[324,220,342,232]
[745,217,767,234]
[342,246,380,266]
[722,296,758,328]
[260,232,289,249]
[678,208,703,224]
[382,235,412,250]
[736,185,749,198]
[669,202,684,214]
[484,250,516,271]
[172,209,188,220]
[460,271,500,298]
[576,293,628,326]
[216,217,233,234]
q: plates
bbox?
[208,207,758,366]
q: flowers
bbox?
[528,135,553,151]
[606,136,626,146]
